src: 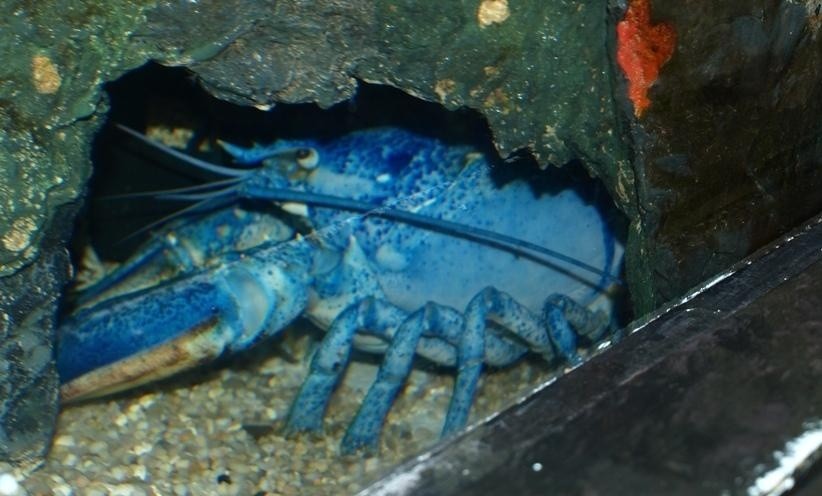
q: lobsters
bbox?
[55,124,625,454]
